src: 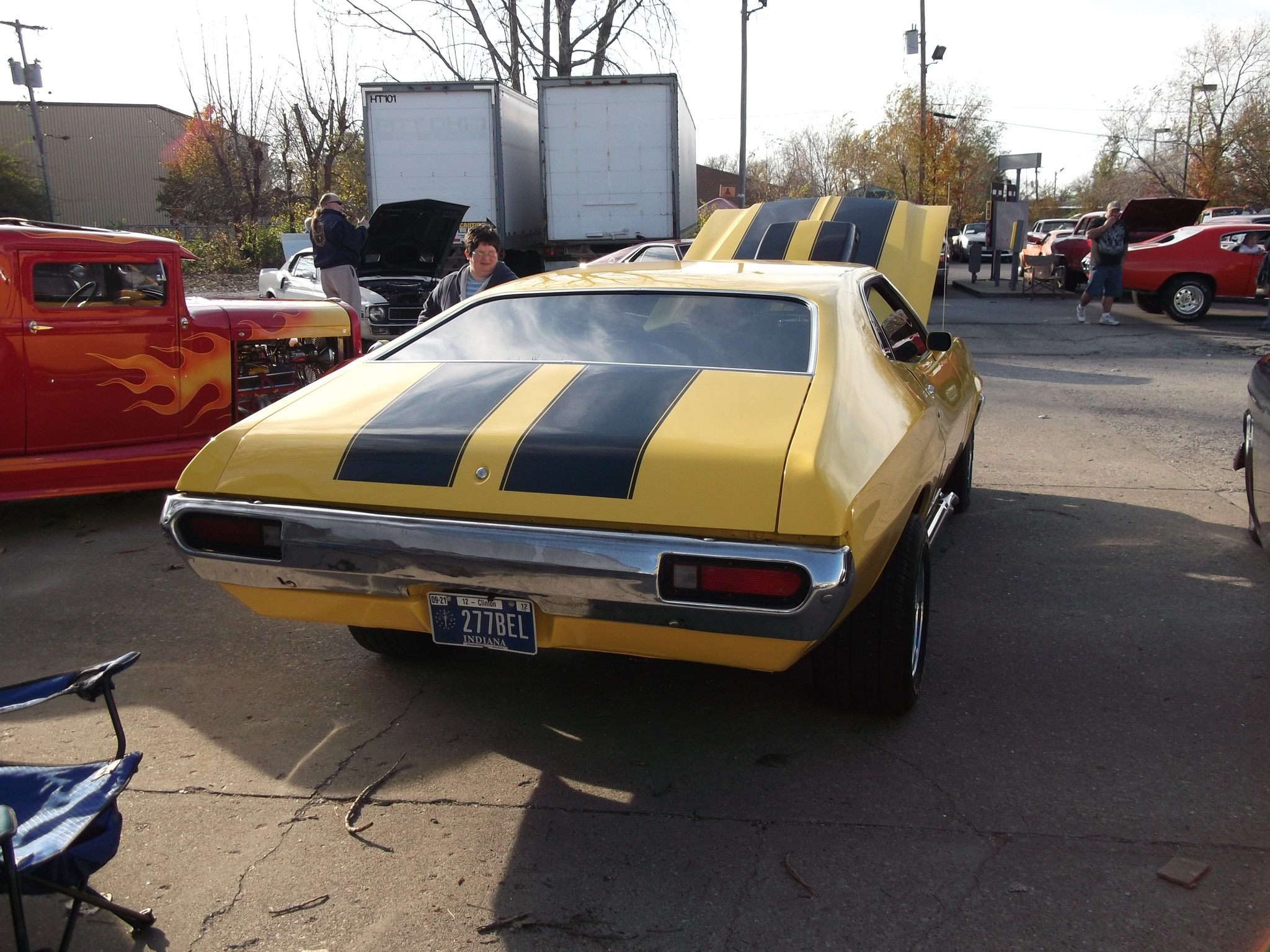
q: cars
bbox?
[0,215,363,504]
[257,198,471,342]
[951,222,1013,264]
[933,227,960,296]
[1232,351,1270,558]
[1018,213,1082,277]
[1198,206,1270,224]
[1082,223,1270,323]
[578,239,695,266]
[1049,197,1210,292]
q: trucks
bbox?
[536,73,700,271]
[357,80,543,279]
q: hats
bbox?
[1107,201,1120,210]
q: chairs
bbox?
[1022,254,1066,301]
[0,650,155,952]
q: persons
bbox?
[1239,231,1264,254]
[1077,201,1129,325]
[417,225,520,326]
[310,193,370,326]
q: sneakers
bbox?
[1077,304,1085,322]
[1100,315,1119,325]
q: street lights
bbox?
[35,133,71,222]
[916,45,947,204]
[737,0,767,209]
[1152,128,1171,198]
[1052,167,1065,218]
[1182,84,1218,198]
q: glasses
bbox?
[326,201,342,205]
[472,251,497,256]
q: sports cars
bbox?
[158,194,985,725]
